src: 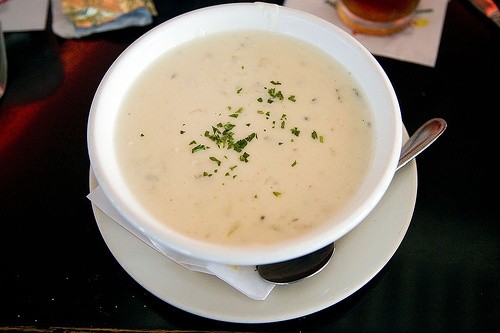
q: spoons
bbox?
[259,118,447,284]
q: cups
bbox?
[338,0,419,35]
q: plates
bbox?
[88,126,416,326]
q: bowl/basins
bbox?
[87,2,403,265]
